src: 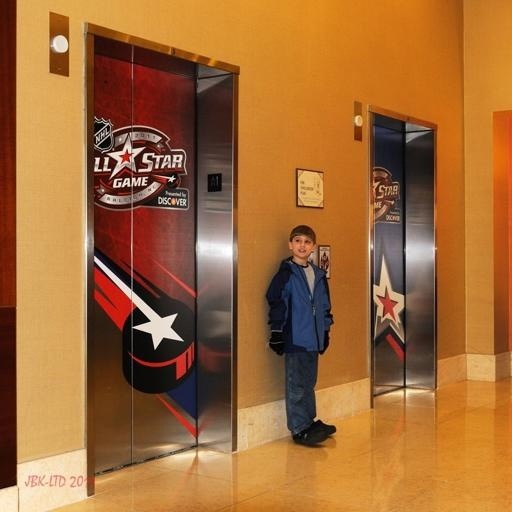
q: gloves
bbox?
[319,331,330,354]
[269,323,283,355]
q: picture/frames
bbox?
[294,168,326,211]
[317,246,331,280]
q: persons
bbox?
[266,224,336,447]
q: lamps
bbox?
[353,115,363,128]
[49,34,69,55]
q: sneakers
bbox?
[293,419,337,445]
